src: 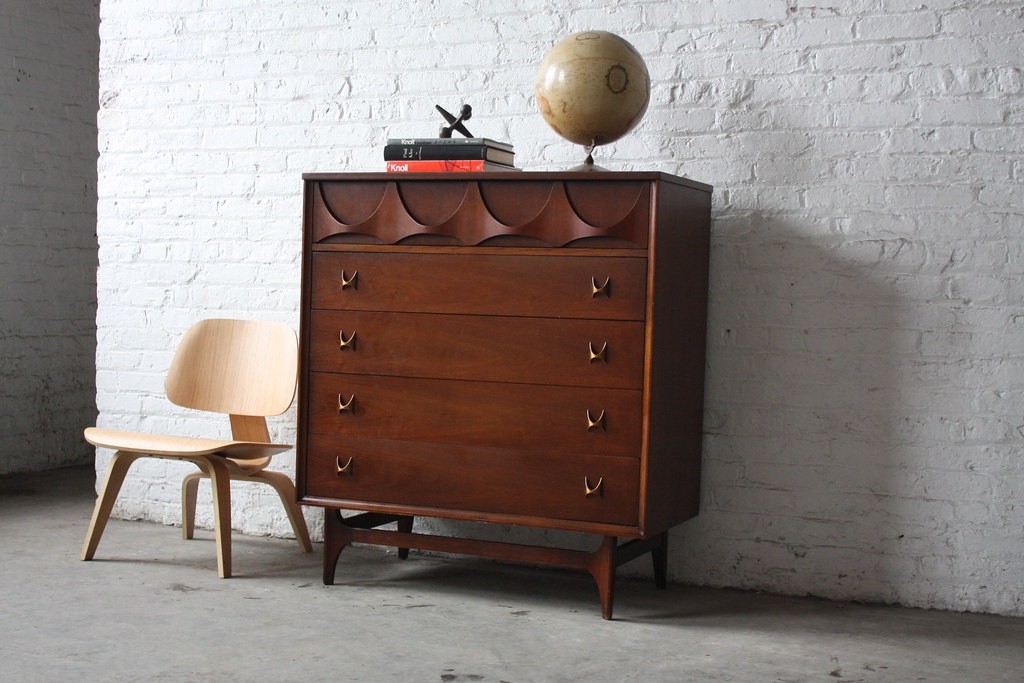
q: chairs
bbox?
[80,318,312,579]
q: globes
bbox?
[535,30,652,172]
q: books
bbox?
[384,137,524,173]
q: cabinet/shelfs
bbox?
[292,172,715,622]
